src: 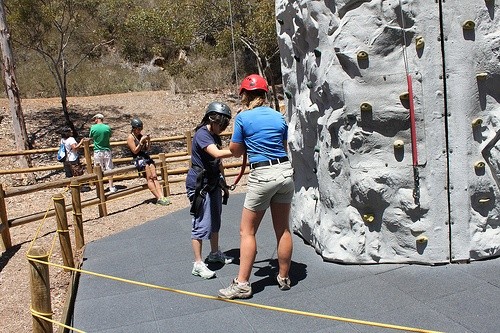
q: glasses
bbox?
[136,128,143,130]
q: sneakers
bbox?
[218,277,252,300]
[208,250,235,264]
[192,261,216,279]
[276,272,291,289]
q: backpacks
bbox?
[57,142,72,162]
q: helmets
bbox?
[206,102,231,118]
[131,118,143,128]
[239,74,268,95]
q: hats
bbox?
[93,114,104,119]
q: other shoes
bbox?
[65,188,71,192]
[157,199,169,205]
[110,187,117,192]
[163,197,172,203]
[81,186,91,192]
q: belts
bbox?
[250,157,288,170]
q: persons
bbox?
[88,113,117,193]
[128,119,172,206]
[186,102,233,278]
[218,74,294,298]
[60,127,91,193]
